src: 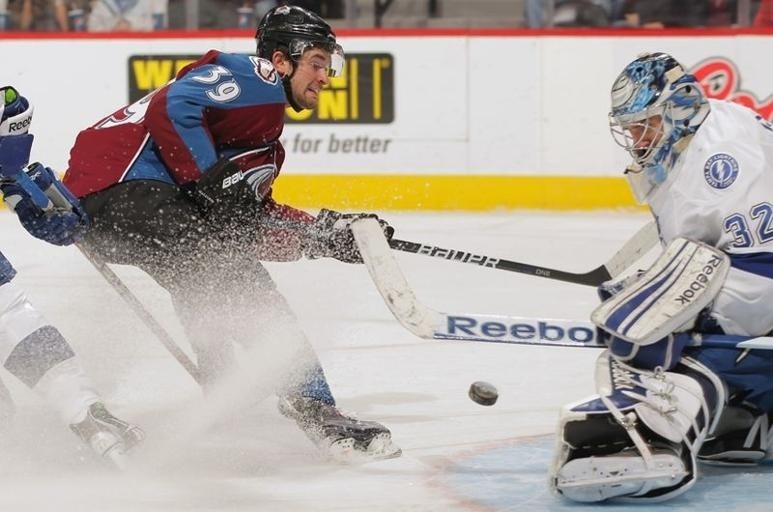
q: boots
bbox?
[275,392,394,461]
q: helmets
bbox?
[606,51,711,203]
[254,4,345,78]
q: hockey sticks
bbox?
[387,220,661,287]
[349,216,773,351]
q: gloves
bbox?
[178,158,263,238]
[0,86,91,247]
[304,208,394,263]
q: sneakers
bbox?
[67,398,138,461]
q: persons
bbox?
[0,83,144,471]
[547,50,773,506]
[523,0,773,35]
[0,0,392,35]
[63,4,398,469]
